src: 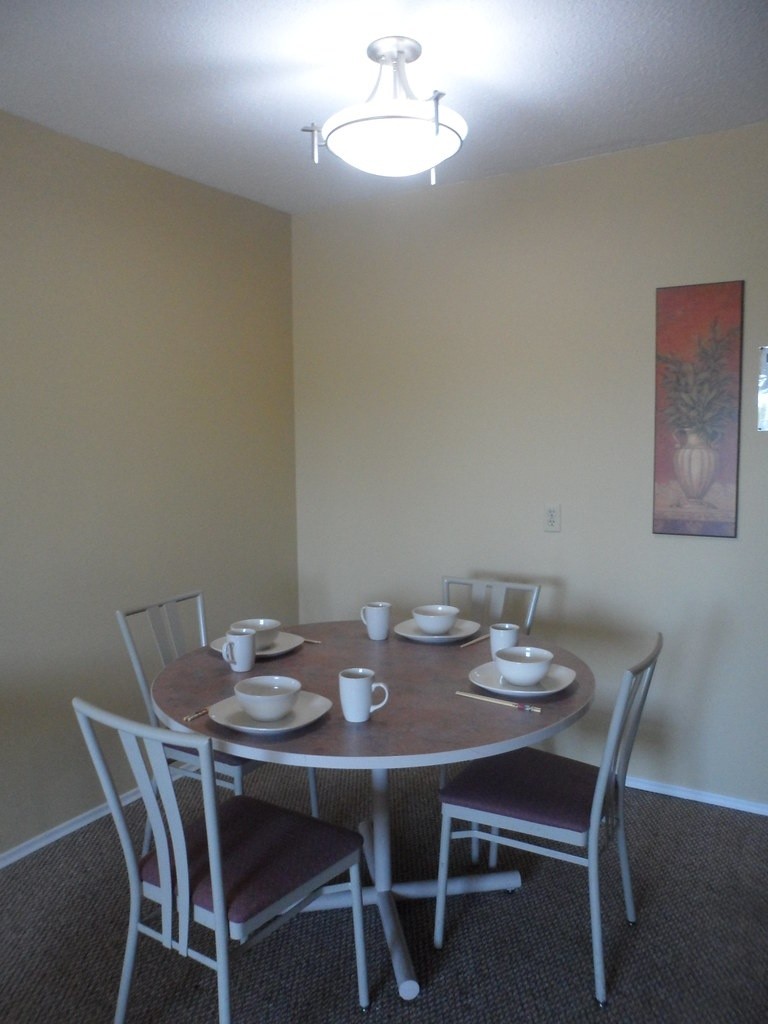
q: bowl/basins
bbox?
[495,647,553,686]
[234,675,301,721]
[412,605,459,634]
[231,619,281,650]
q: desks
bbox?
[145,613,602,1001]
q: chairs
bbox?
[426,577,545,790]
[112,588,321,858]
[423,632,668,1008]
[70,695,375,1024]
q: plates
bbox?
[394,619,480,642]
[211,632,304,657]
[208,691,332,733]
[469,661,576,697]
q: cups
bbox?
[490,623,520,661]
[338,668,388,723]
[222,628,256,672]
[360,602,392,640]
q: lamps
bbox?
[300,33,471,188]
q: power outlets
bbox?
[543,502,561,533]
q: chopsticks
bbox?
[456,691,541,713]
[460,634,489,647]
[184,708,207,722]
[305,639,321,644]
